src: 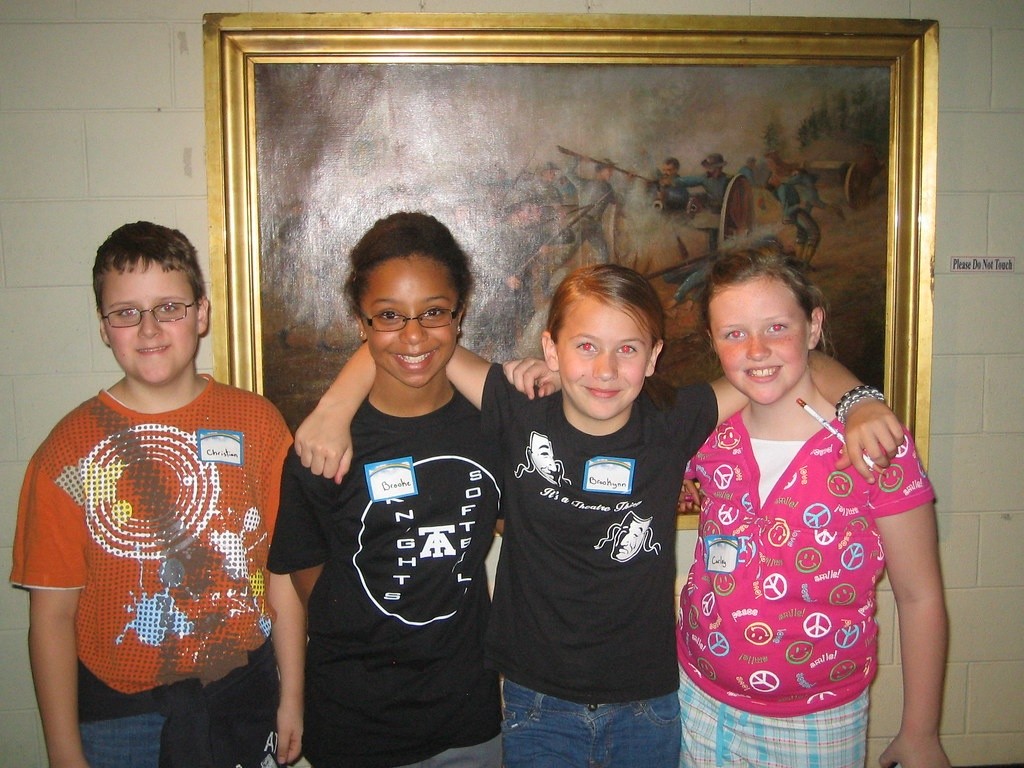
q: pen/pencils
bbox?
[797,395,891,483]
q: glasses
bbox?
[102,296,199,328]
[360,299,465,332]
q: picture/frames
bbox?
[202,11,941,536]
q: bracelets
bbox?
[835,385,886,425]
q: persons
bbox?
[267,208,703,768]
[502,237,953,768]
[296,265,906,768]
[9,221,305,767]
